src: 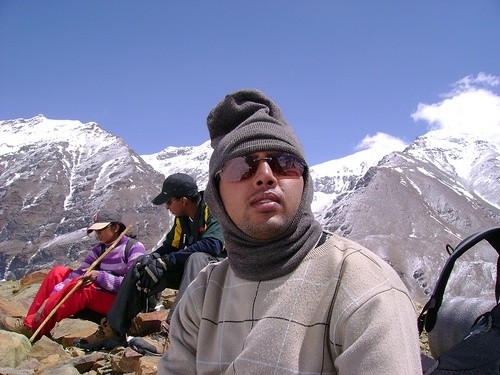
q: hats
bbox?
[203,87,321,281]
[152,173,197,206]
[86,209,120,235]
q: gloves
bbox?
[135,258,169,293]
[135,252,162,280]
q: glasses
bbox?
[215,155,306,183]
[165,198,179,206]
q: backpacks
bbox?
[417,226,500,375]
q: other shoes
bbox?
[2,316,35,337]
[73,317,129,350]
[129,326,168,357]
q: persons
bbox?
[1,208,148,343]
[73,172,228,350]
[156,89,422,375]
